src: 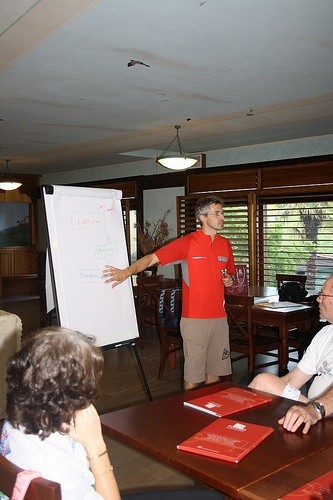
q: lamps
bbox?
[0,159,23,191]
[155,124,197,171]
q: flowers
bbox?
[136,216,170,255]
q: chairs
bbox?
[136,273,310,382]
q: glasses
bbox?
[319,291,333,299]
[201,212,224,218]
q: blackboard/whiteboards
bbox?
[41,184,143,352]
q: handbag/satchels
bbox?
[278,282,308,303]
[224,265,248,295]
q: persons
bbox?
[102,197,236,389]
[247,274,333,434]
[0,326,122,500]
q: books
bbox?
[177,418,275,463]
[256,301,302,308]
[184,386,273,418]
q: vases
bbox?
[148,263,159,278]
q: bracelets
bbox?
[94,466,113,477]
[231,274,236,281]
[87,451,107,460]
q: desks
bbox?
[97,383,333,500]
[227,284,314,379]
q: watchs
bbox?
[311,401,325,419]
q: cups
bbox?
[144,270,152,277]
